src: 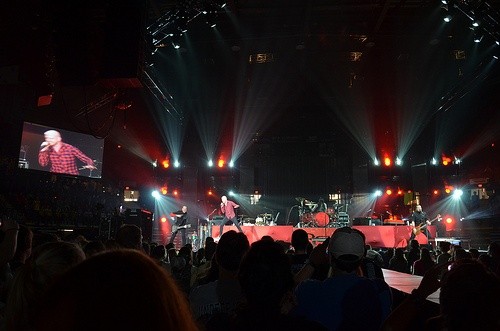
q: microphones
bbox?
[40,145,46,150]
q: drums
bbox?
[303,208,335,227]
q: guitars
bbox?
[412,213,442,235]
[171,224,192,233]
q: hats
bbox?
[330,227,364,263]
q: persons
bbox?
[1,221,499,331]
[407,204,430,244]
[319,199,327,212]
[433,213,447,239]
[216,195,244,239]
[470,190,481,203]
[166,206,189,249]
[38,129,96,175]
